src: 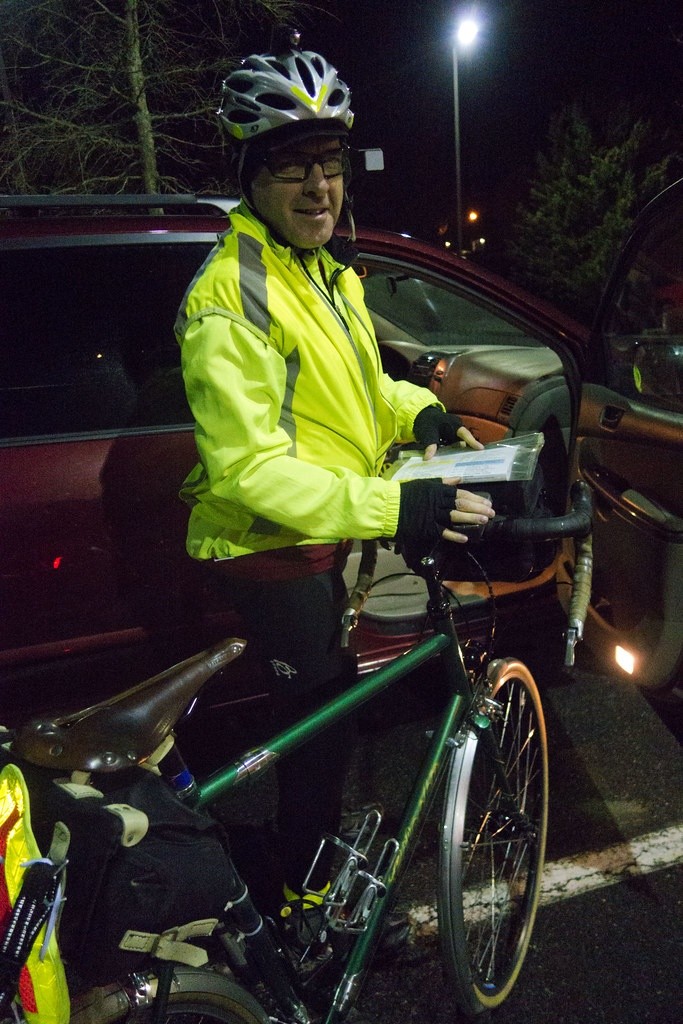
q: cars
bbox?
[0,193,682,734]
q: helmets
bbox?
[214,27,355,158]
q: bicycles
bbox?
[0,478,598,1024]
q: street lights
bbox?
[448,1,492,254]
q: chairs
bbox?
[66,339,137,431]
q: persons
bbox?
[177,50,496,968]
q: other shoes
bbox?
[276,896,414,975]
[336,800,385,843]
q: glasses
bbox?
[252,142,353,180]
